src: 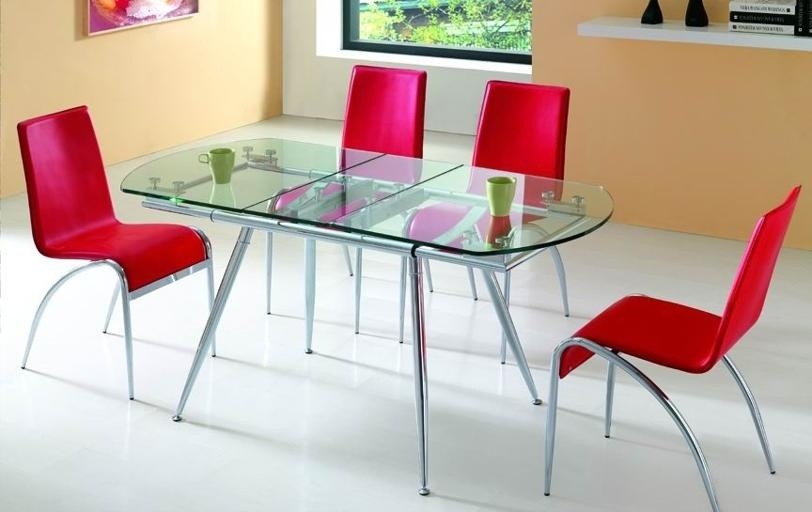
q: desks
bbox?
[121,138,616,497]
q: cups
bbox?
[209,148,234,184]
[486,176,516,217]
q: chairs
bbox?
[400,80,571,366]
[268,64,427,355]
[544,184,801,511]
[16,104,217,402]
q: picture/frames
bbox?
[87,1,200,38]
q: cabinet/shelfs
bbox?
[560,17,812,249]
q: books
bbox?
[729,0,812,36]
